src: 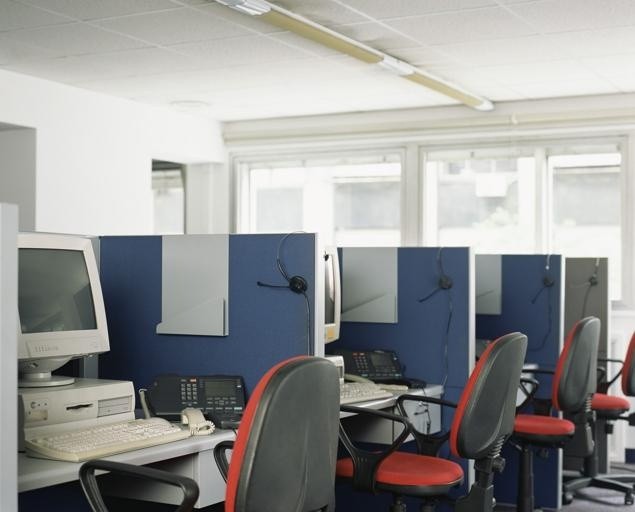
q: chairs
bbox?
[562,331,635,505]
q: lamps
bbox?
[214,0,494,111]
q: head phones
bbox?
[544,254,554,287]
[589,257,600,286]
[436,248,452,289]
[277,232,307,294]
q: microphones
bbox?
[417,287,438,302]
[257,281,289,289]
[532,287,543,305]
[568,282,590,288]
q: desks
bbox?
[0,202,611,512]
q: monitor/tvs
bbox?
[325,247,341,357]
[17,233,112,387]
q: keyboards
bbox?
[25,418,190,464]
[339,382,393,405]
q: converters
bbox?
[212,414,241,429]
[400,378,426,388]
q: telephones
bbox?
[181,407,208,436]
[344,373,375,385]
